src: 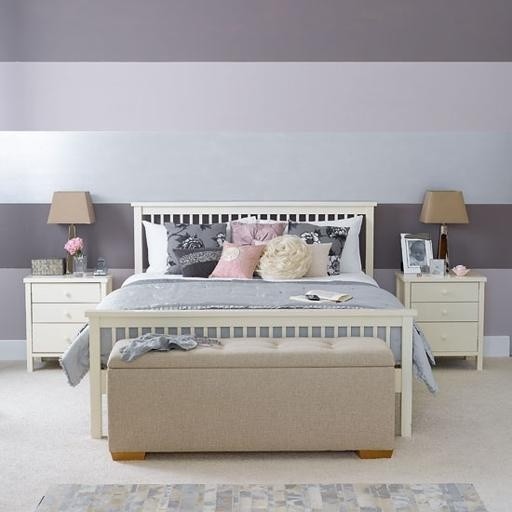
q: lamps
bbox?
[46,189,97,274]
[418,188,470,273]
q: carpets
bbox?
[31,479,487,512]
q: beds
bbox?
[84,197,420,444]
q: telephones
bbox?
[93,256,108,276]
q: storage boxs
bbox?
[31,257,67,276]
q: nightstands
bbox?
[392,269,488,371]
[21,270,114,375]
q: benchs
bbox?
[105,335,398,464]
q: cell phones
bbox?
[308,295,319,301]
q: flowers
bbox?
[64,237,88,258]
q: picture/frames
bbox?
[399,230,435,274]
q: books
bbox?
[288,289,353,303]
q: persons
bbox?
[409,241,425,266]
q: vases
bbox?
[71,255,88,274]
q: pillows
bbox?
[140,215,368,278]
